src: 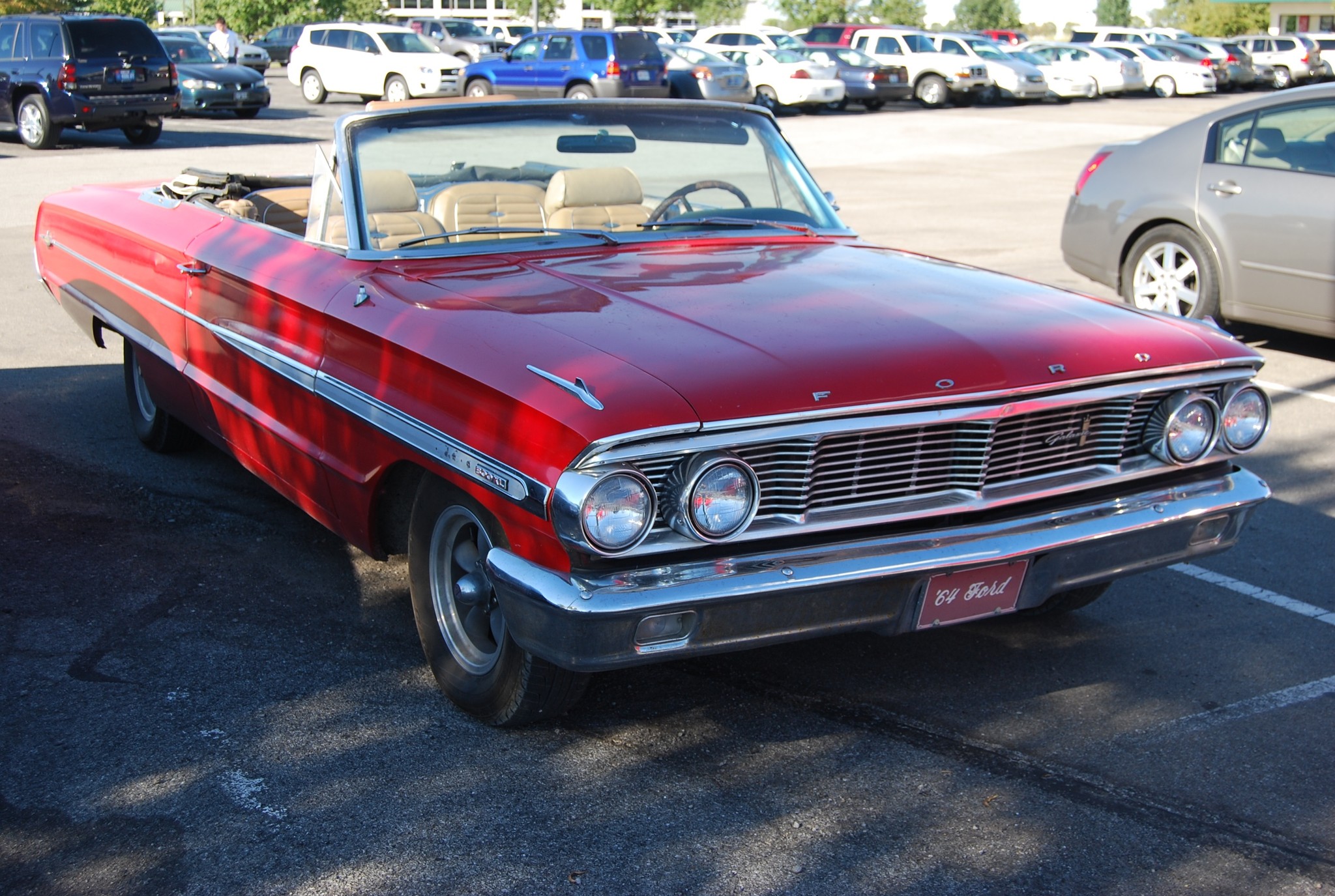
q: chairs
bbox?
[545,42,571,58]
[306,166,450,249]
[242,187,320,236]
[426,181,546,242]
[543,170,657,235]
[1224,128,1294,171]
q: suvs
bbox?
[0,10,180,150]
[402,12,1335,126]
[250,26,327,65]
[155,25,272,75]
[287,21,467,104]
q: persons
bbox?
[207,18,239,64]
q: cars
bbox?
[1061,83,1335,342]
[151,37,272,120]
[33,100,1274,733]
